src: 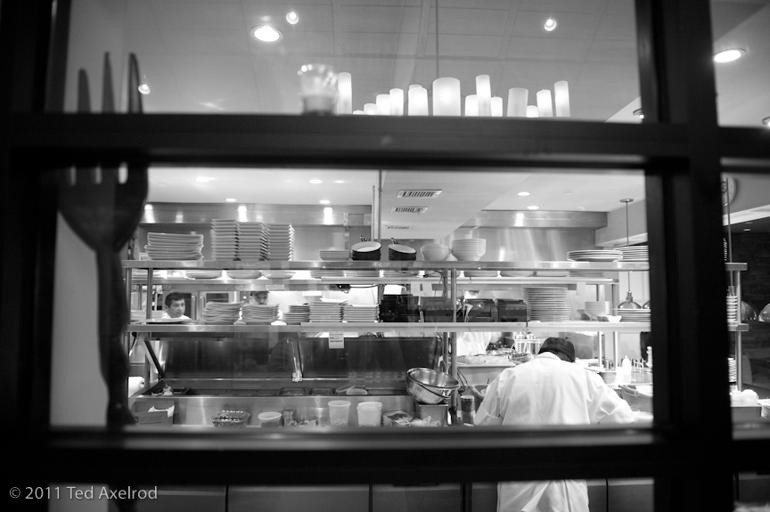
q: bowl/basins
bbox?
[405,366,460,404]
[388,237,487,261]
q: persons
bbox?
[161,292,192,320]
[250,291,269,305]
[474,338,636,512]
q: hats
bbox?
[540,337,575,362]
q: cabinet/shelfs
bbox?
[124,260,752,398]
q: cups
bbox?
[258,411,281,428]
[327,398,351,427]
[357,400,383,427]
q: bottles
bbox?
[621,355,632,370]
[460,389,477,423]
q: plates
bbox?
[144,218,380,324]
[527,245,738,322]
